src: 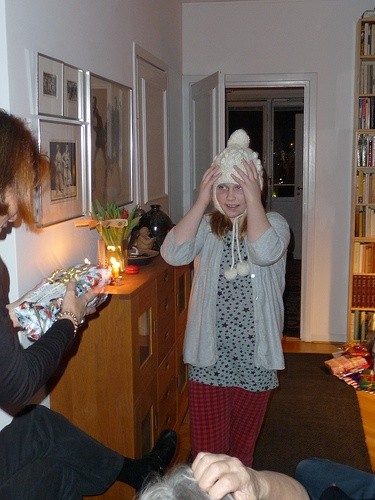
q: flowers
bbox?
[90,198,142,249]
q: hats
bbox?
[210,128,264,218]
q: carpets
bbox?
[253,353,373,478]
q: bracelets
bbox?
[54,311,79,337]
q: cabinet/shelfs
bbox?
[347,16,375,345]
[49,256,191,500]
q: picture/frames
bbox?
[37,52,63,117]
[63,61,84,126]
[86,69,134,216]
[37,118,86,228]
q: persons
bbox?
[138,451,375,500]
[160,129,290,469]
[0,107,178,500]
[53,144,73,196]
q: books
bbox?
[353,241,375,274]
[357,132,375,167]
[360,21,375,56]
[351,310,375,341]
[359,97,375,129]
[352,275,375,308]
[356,170,375,204]
[355,206,375,237]
[360,60,375,95]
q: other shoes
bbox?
[136,431,178,489]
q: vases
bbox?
[105,246,125,273]
